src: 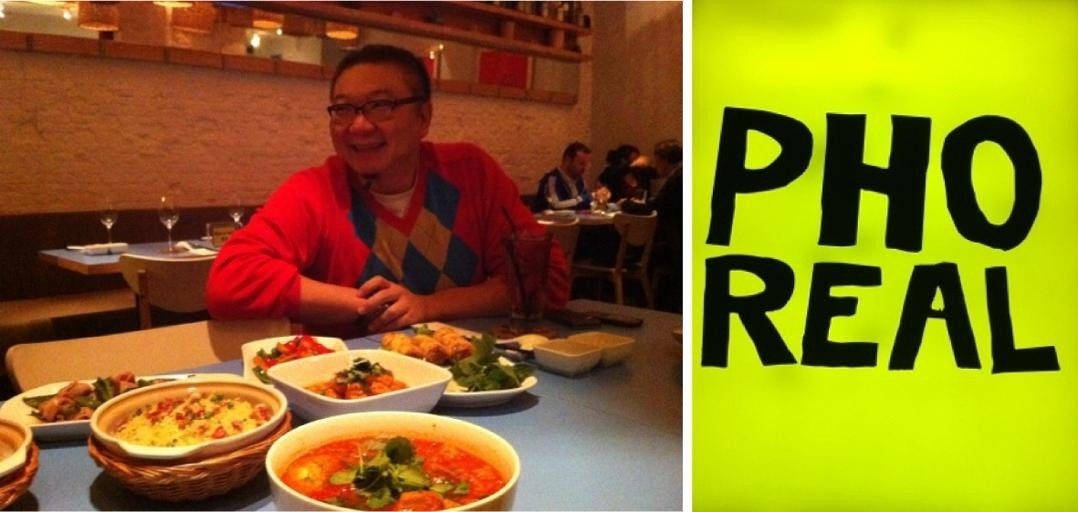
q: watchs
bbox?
[341,302,373,336]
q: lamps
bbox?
[249,10,284,30]
[78,2,123,31]
[322,22,362,41]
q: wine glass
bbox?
[157,195,179,254]
[96,196,122,245]
[229,207,245,228]
[540,183,556,219]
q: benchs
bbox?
[0,286,138,344]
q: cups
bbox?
[507,230,554,338]
[592,182,609,217]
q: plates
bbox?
[534,331,635,378]
[81,243,128,255]
[5,334,540,512]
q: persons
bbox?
[617,137,682,306]
[534,141,611,266]
[205,46,569,336]
[600,144,659,197]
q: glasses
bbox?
[326,93,422,122]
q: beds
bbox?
[0,297,681,512]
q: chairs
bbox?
[6,320,308,390]
[116,254,217,327]
[539,218,580,256]
[570,211,659,309]
